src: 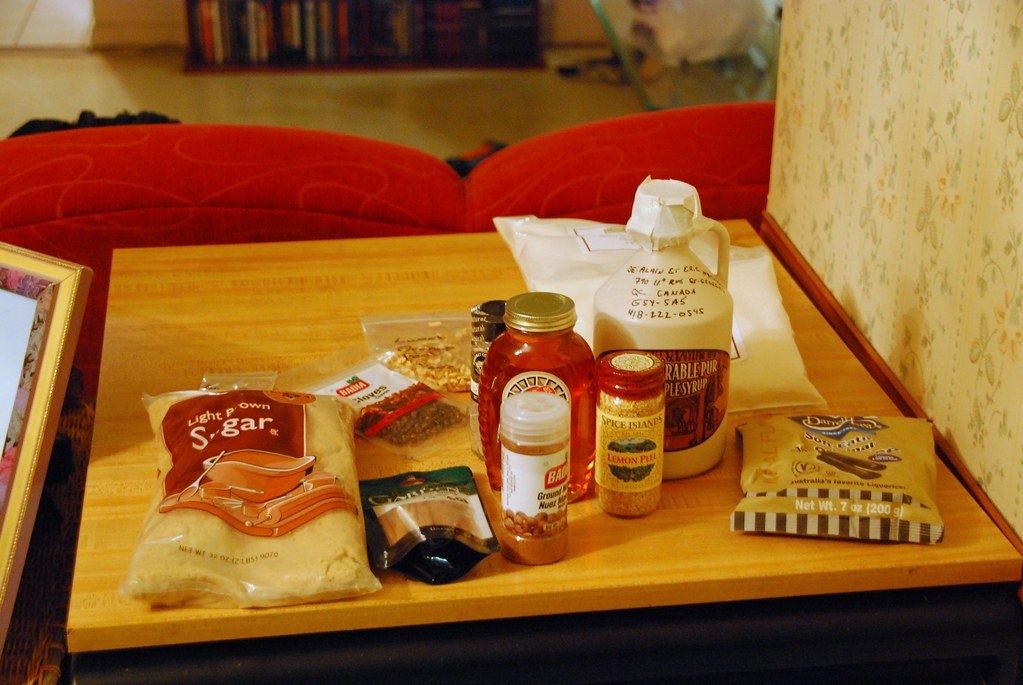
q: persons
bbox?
[629,0,776,83]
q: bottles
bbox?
[592,178,735,483]
[469,299,596,505]
[498,393,571,567]
[595,349,666,517]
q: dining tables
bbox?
[66,219,1022,685]
